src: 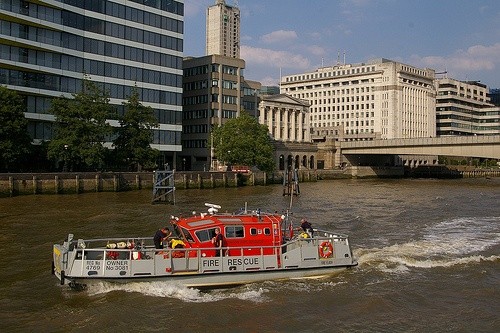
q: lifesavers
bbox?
[320,242,332,258]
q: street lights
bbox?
[226,150,232,172]
[63,145,69,173]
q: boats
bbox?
[51,201,359,290]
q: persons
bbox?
[296,218,314,244]
[153,227,173,255]
[212,227,225,257]
